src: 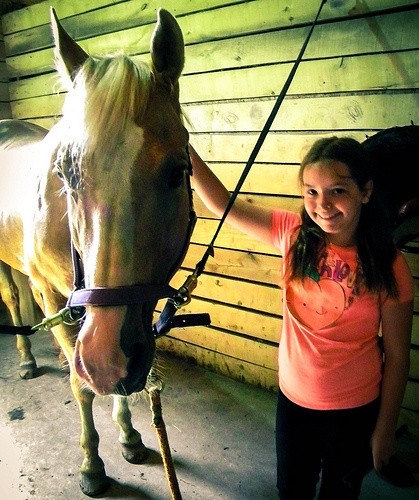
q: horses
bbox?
[1,6,197,495]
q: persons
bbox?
[187,136,416,500]
